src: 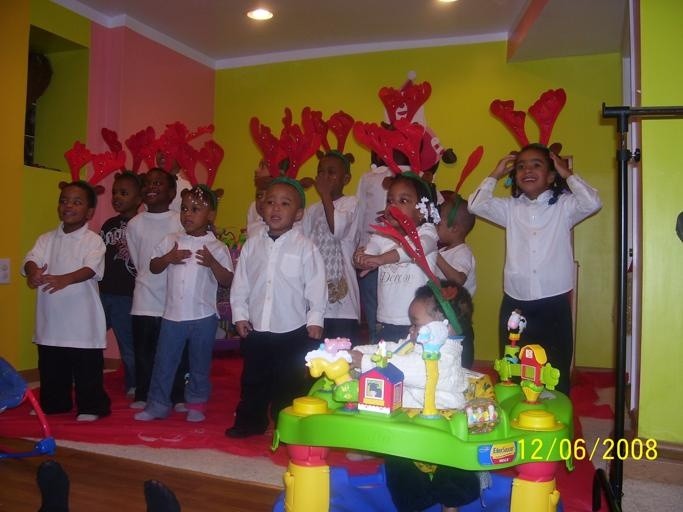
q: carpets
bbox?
[0,355,682,512]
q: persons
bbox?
[245,156,271,231]
[16,179,112,426]
[247,176,280,237]
[123,167,188,414]
[350,171,436,341]
[355,145,432,343]
[437,189,478,374]
[465,143,604,393]
[131,183,234,424]
[303,150,361,344]
[221,174,325,440]
[383,278,482,512]
[95,167,145,399]
